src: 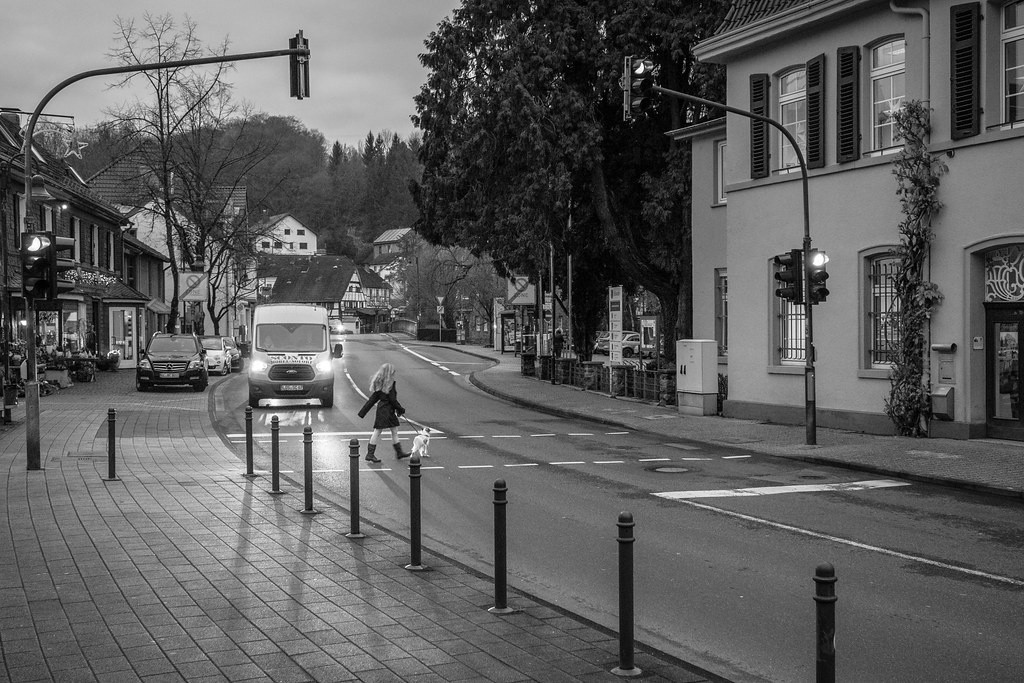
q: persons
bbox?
[357,363,412,462]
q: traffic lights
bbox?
[623,55,654,115]
[21,228,78,301]
[776,249,830,306]
[288,38,310,99]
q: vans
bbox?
[239,301,344,407]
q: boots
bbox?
[365,443,381,463]
[393,442,411,459]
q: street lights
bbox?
[406,254,420,307]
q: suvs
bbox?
[137,331,210,391]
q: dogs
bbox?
[409,427,431,459]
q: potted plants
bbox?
[45,365,69,388]
[4,378,22,405]
[80,365,93,381]
[13,355,21,365]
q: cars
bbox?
[553,327,668,362]
[222,337,244,372]
[329,319,345,336]
[198,336,231,376]
